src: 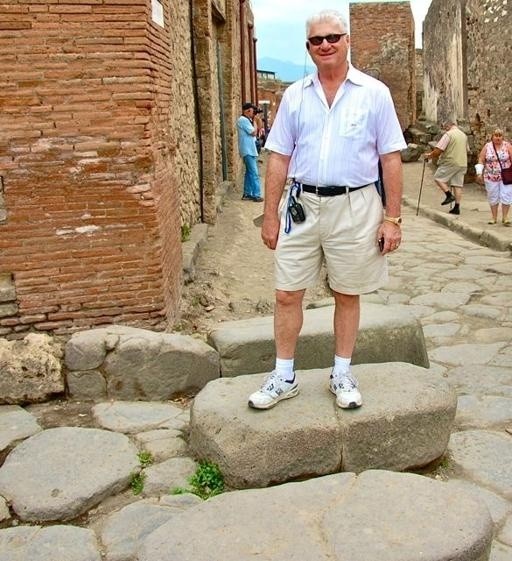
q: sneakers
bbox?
[252,197,264,203]
[327,366,363,411]
[247,368,301,411]
[501,219,512,226]
[239,194,253,200]
[440,195,456,206]
[488,218,497,226]
[448,208,460,215]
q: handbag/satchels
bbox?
[501,168,512,185]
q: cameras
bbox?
[254,108,263,115]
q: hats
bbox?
[242,102,256,110]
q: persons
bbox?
[475,128,512,228]
[424,119,468,215]
[249,12,406,411]
[236,102,272,202]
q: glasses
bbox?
[305,32,348,47]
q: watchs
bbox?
[384,214,401,226]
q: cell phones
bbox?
[378,238,384,251]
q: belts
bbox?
[292,179,371,198]
